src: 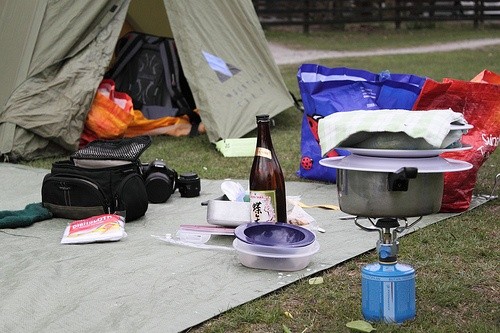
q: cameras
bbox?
[140,159,177,204]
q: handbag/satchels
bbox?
[43,136,155,222]
[294,62,425,182]
[413,68,500,212]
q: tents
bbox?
[0,0,294,163]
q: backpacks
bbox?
[106,30,199,129]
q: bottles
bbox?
[249,114,287,223]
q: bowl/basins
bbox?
[345,129,470,149]
[234,222,316,248]
[202,193,301,226]
[233,237,321,272]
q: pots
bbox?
[336,166,445,217]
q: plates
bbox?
[319,153,474,173]
[331,143,474,158]
[450,123,474,130]
